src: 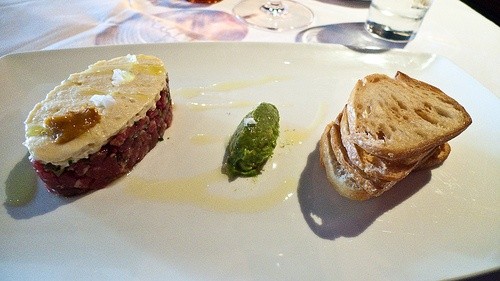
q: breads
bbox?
[319,72,472,202]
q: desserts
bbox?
[20,53,173,198]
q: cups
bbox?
[364,0,432,43]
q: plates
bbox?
[232,0,315,31]
[0,42,499,281]
